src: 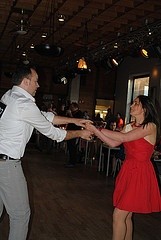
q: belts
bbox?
[0,154,17,160]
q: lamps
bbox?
[110,53,128,66]
[15,10,28,35]
[57,12,65,22]
[140,42,160,59]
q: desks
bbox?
[79,138,97,165]
[98,143,120,177]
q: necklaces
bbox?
[133,123,142,128]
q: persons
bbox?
[47,103,57,115]
[106,108,121,133]
[0,65,93,240]
[85,95,161,240]
[64,102,82,168]
